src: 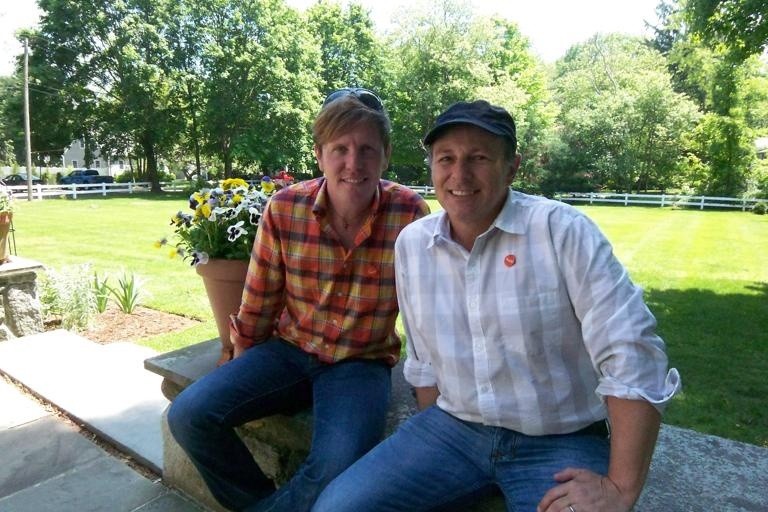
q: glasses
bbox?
[321,89,383,111]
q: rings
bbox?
[567,504,575,512]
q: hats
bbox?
[424,100,516,151]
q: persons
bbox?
[309,100,684,512]
[166,88,431,510]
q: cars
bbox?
[0,172,46,193]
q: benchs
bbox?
[142,336,767,511]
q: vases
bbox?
[195,259,250,367]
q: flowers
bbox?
[154,170,296,269]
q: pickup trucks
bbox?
[59,169,115,192]
